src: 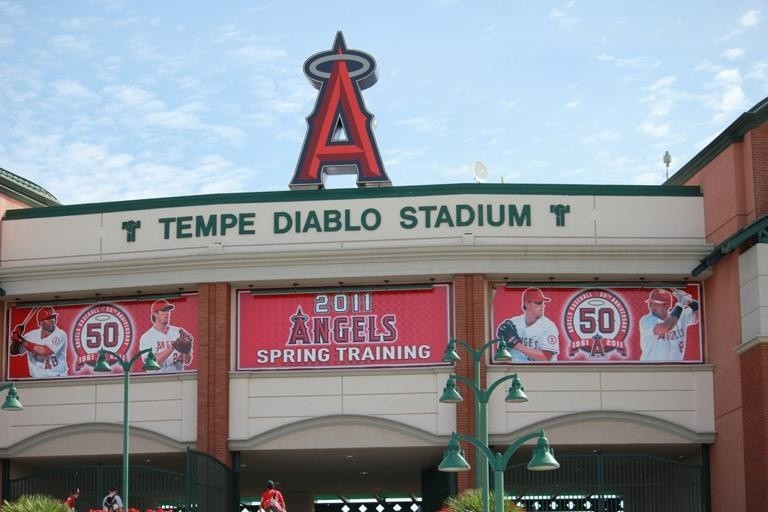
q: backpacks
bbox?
[104,494,119,512]
[264,491,286,512]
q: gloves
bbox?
[674,290,693,307]
[11,324,24,343]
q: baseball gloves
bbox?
[496,319,522,349]
[174,328,194,355]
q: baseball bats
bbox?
[20,306,39,327]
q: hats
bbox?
[151,300,175,315]
[523,288,551,304]
[644,288,672,305]
[265,480,274,487]
[37,307,58,323]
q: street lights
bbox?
[437,336,560,512]
[93,347,161,512]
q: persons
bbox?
[102,485,123,512]
[64,487,79,512]
[637,287,699,362]
[258,481,285,511]
[9,306,68,379]
[138,298,192,371]
[494,286,560,362]
